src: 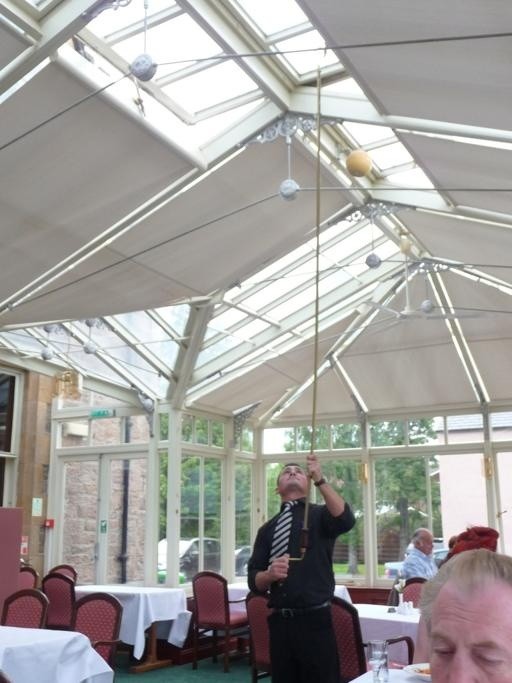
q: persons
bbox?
[402,527,439,597]
[248,456,354,683]
[419,550,512,683]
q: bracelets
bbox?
[314,477,325,486]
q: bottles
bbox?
[402,600,413,613]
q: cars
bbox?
[157,536,220,579]
[233,545,251,576]
[383,549,450,580]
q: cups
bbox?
[368,640,389,683]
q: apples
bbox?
[347,149,372,177]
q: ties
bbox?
[266,498,301,587]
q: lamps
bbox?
[329,144,373,178]
[397,230,412,253]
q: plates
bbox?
[403,662,433,681]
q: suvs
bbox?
[403,535,444,559]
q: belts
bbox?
[273,598,334,619]
